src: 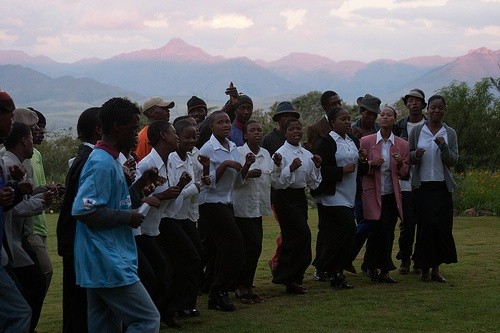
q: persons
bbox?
[220,82,460,303]
[56,96,256,333]
[0,90,62,333]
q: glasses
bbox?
[189,108,205,113]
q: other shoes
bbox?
[286,282,309,294]
[326,271,353,289]
[208,289,241,312]
[313,268,327,281]
[361,261,369,272]
[421,270,432,282]
[398,256,412,274]
[431,272,448,282]
[177,304,201,316]
[161,306,181,328]
[343,263,357,274]
[412,264,422,273]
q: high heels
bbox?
[235,284,265,304]
[366,270,400,283]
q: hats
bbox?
[403,89,427,110]
[186,96,207,114]
[357,94,382,115]
[142,97,175,112]
[271,102,300,123]
[0,92,16,116]
[12,107,39,127]
[235,95,253,108]
